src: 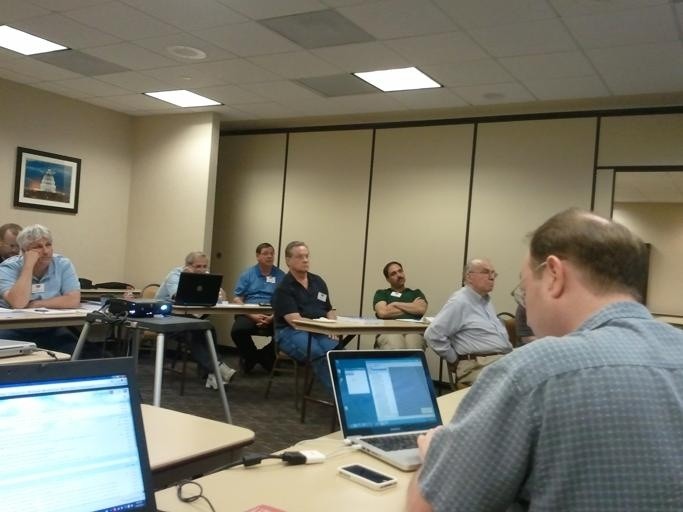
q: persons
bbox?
[514,303,537,344]
[154,252,237,390]
[0,223,81,354]
[270,240,345,400]
[0,222,24,259]
[231,242,286,376]
[423,258,514,386]
[373,261,428,352]
[406,207,683,512]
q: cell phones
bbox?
[337,463,398,488]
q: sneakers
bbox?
[206,361,237,390]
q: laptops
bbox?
[0,338,37,358]
[0,356,157,512]
[326,349,443,472]
[165,272,223,306]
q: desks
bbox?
[1,287,455,432]
[140,403,256,492]
[154,385,472,512]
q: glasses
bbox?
[511,262,547,309]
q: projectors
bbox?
[108,298,171,318]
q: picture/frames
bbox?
[14,145,81,212]
